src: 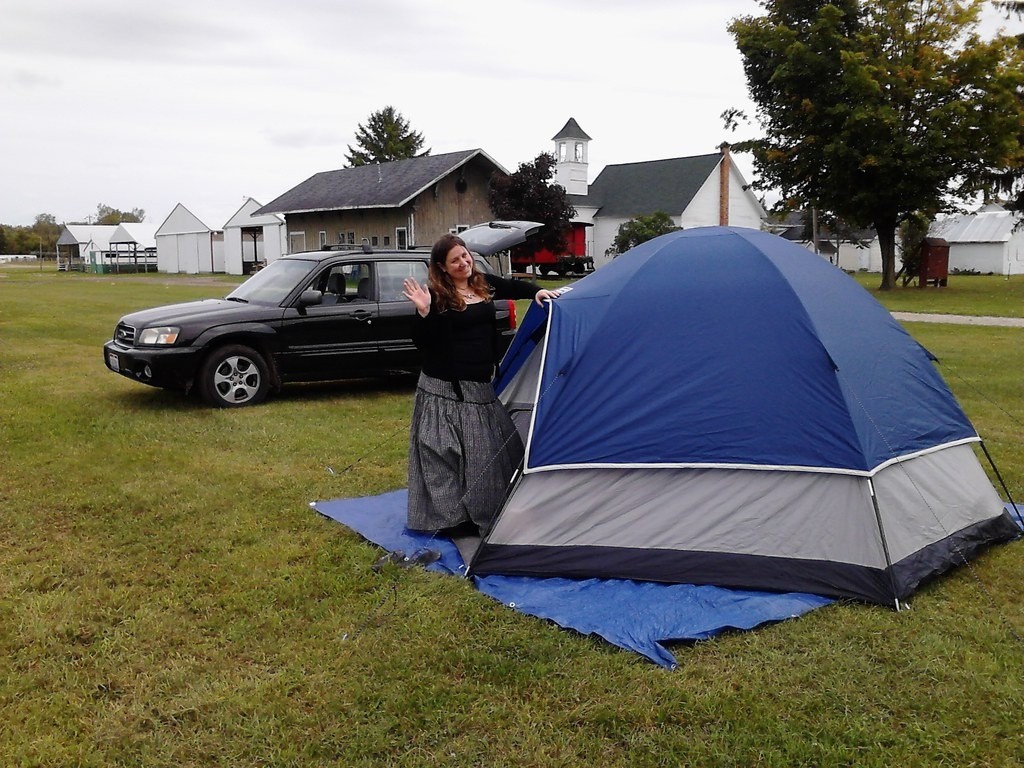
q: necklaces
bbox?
[462,294,477,299]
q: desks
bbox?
[507,273,533,282]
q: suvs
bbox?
[102,220,546,408]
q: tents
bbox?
[55,196,287,276]
[458,224,1024,613]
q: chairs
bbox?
[350,278,368,302]
[321,273,348,304]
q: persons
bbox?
[397,233,563,539]
